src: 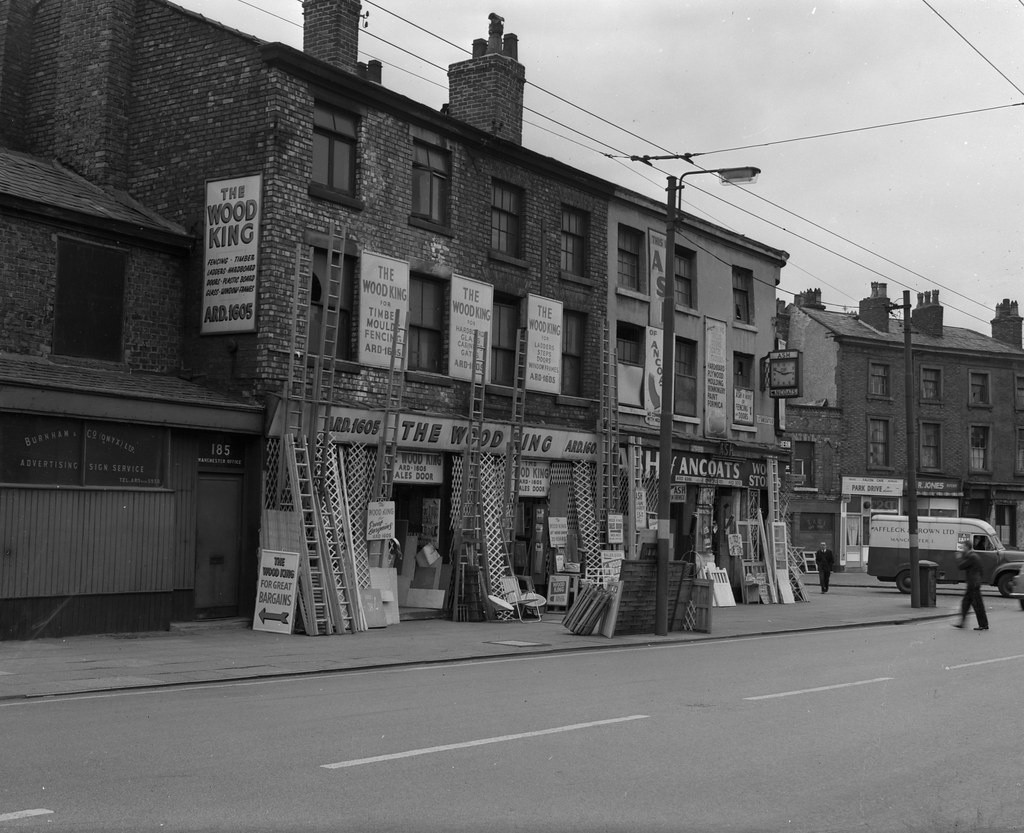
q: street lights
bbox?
[656,167,761,636]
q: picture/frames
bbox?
[647,227,667,331]
[524,293,562,395]
[199,171,265,336]
[447,272,492,385]
[358,250,410,371]
[733,386,757,426]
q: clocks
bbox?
[769,349,802,397]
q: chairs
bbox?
[500,576,542,623]
[487,594,514,622]
[520,592,547,622]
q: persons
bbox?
[816,542,833,594]
[953,540,989,630]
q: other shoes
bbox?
[953,624,965,628]
[973,626,989,631]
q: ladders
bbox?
[597,320,620,553]
[767,458,781,525]
[367,309,411,566]
[457,329,493,598]
[500,328,529,577]
[284,218,356,636]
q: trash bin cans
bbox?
[918,559,940,607]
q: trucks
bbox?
[868,515,1024,595]
